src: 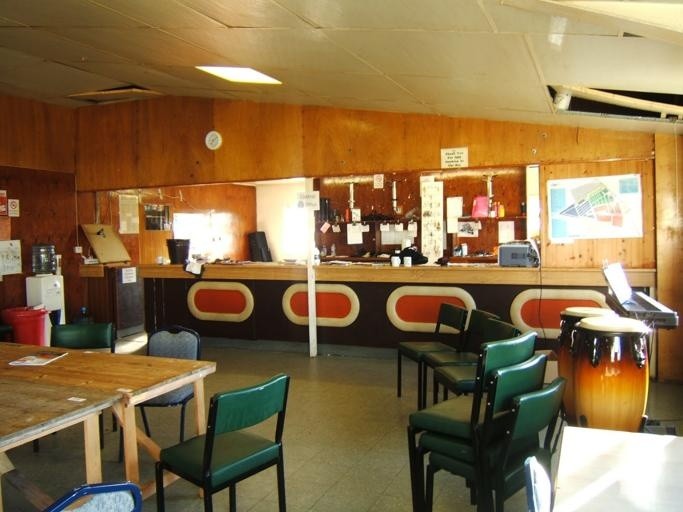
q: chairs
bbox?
[32,321,118,455]
[152,372,290,512]
[43,481,140,512]
[117,327,201,462]
[393,303,566,512]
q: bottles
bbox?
[314,254,321,266]
[321,243,336,256]
[488,201,505,218]
[329,208,352,223]
[461,243,468,258]
[521,201,526,216]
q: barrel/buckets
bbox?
[3,307,47,345]
[32,244,56,274]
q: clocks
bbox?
[205,130,222,151]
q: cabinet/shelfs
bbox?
[103,184,256,262]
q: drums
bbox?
[575,317,649,430]
[557,306,620,426]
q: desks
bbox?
[546,425,680,511]
[0,337,217,506]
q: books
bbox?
[8,351,68,366]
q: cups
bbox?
[390,256,401,268]
[155,257,172,265]
[402,256,412,268]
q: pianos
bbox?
[608,291,678,328]
